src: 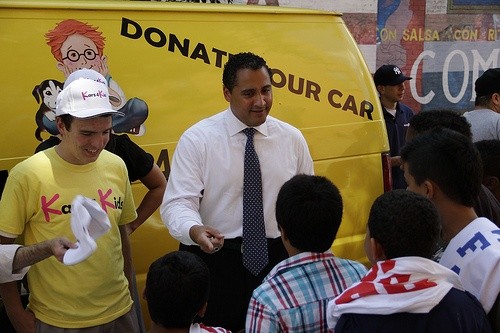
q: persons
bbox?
[145,250,231,333]
[326,190,492,333]
[399,126,500,333]
[34,68,167,333]
[160,52,314,333]
[0,77,138,333]
[474,139,500,206]
[0,237,78,283]
[245,174,370,333]
[406,110,500,229]
[461,68,500,142]
[374,64,415,190]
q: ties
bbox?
[241,128,269,275]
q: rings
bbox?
[213,246,219,252]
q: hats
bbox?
[62,68,120,103]
[475,68,500,95]
[55,78,125,119]
[63,196,111,264]
[374,63,412,87]
